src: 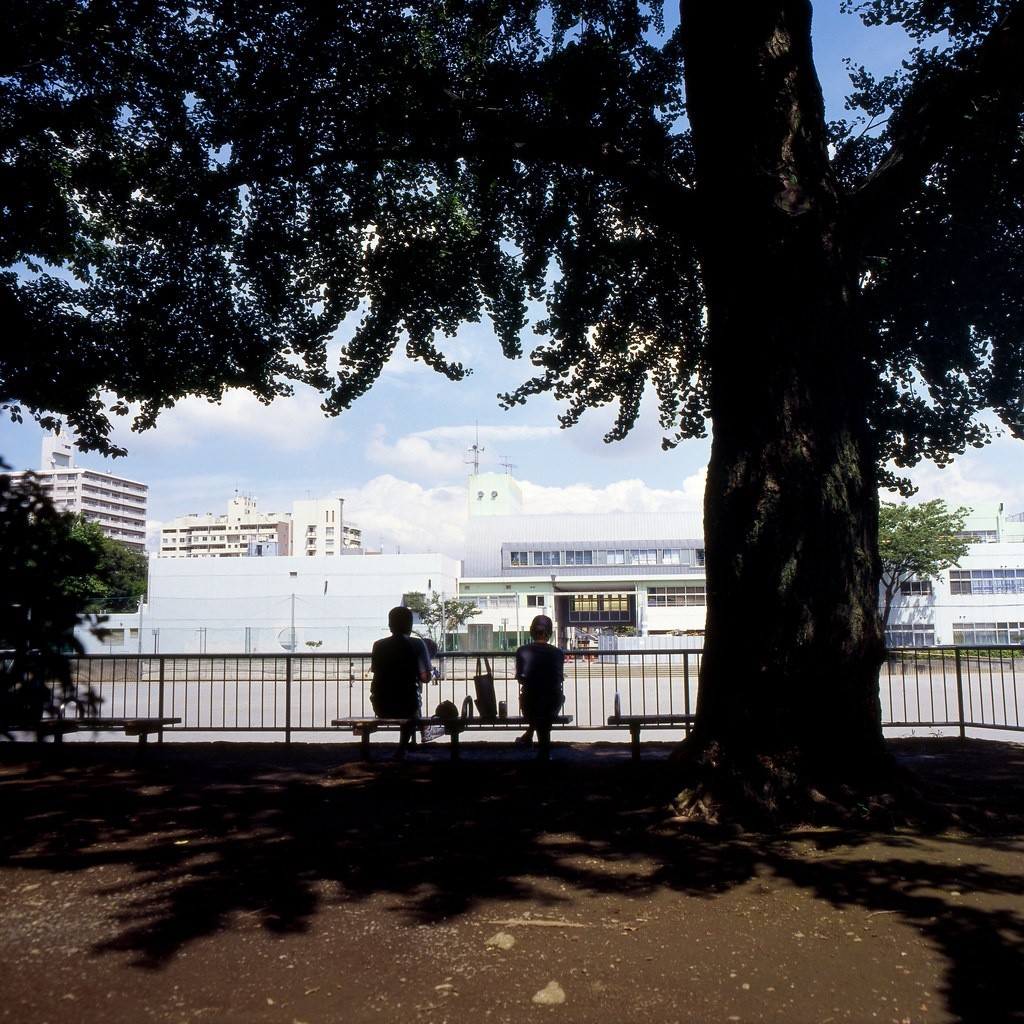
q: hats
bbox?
[532,615,552,635]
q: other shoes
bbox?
[533,741,551,750]
[408,742,417,752]
[394,750,408,761]
[516,736,532,745]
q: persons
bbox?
[351,663,356,687]
[432,667,440,685]
[514,616,565,761]
[370,606,445,763]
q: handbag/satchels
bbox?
[473,656,497,717]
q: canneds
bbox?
[499,701,507,717]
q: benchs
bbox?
[331,716,576,771]
[607,692,697,759]
[28,714,181,757]
